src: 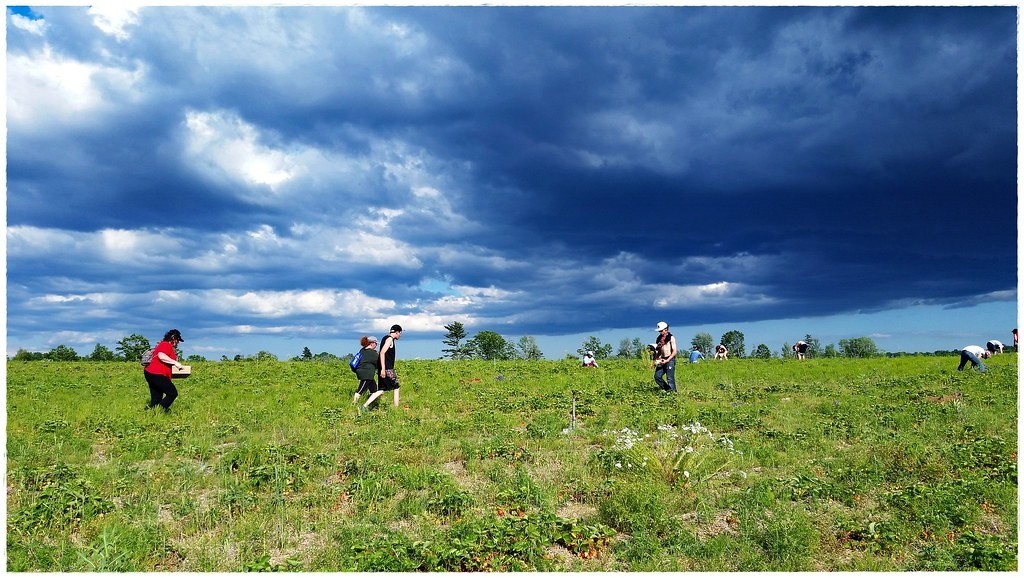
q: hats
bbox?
[390,324,405,332]
[168,329,185,342]
[655,321,668,332]
[587,351,594,356]
[367,336,379,344]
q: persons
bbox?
[987,340,1006,355]
[792,341,807,360]
[715,345,728,360]
[689,346,706,364]
[649,322,677,393]
[357,324,402,418]
[582,351,599,368]
[141,329,185,414]
[957,346,990,373]
[1011,328,1018,352]
[352,336,379,406]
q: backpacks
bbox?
[141,347,155,366]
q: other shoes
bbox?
[361,405,367,414]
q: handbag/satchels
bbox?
[349,352,363,368]
